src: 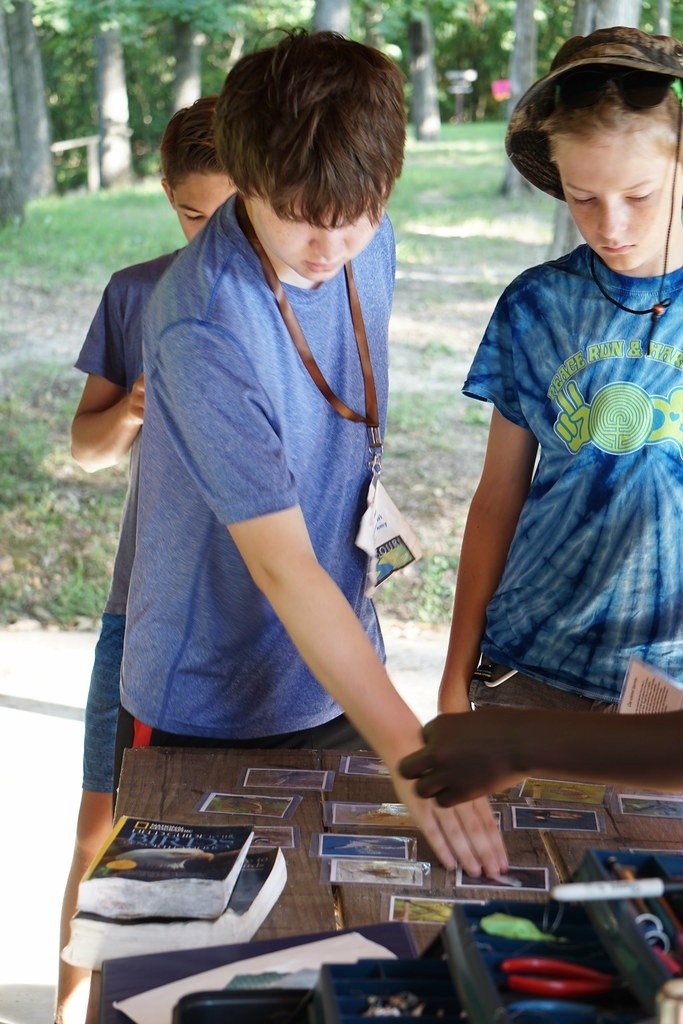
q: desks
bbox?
[80,746,683,1024]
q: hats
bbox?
[505,26,683,204]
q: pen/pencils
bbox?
[549,876,683,903]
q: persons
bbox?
[397,704,683,808]
[54,96,244,1024]
[110,31,512,879]
[438,28,683,716]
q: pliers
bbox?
[499,956,631,996]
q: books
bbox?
[58,815,288,972]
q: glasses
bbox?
[555,66,677,109]
